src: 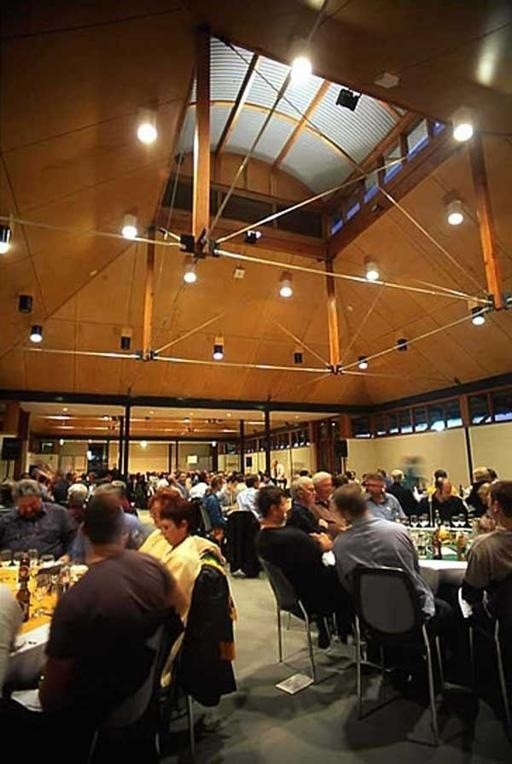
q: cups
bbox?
[451,516,460,530]
[32,574,49,619]
[0,550,12,567]
[468,514,481,537]
[419,513,428,527]
[411,515,418,528]
[50,563,71,608]
[40,554,55,568]
[27,549,37,565]
[394,516,409,526]
[458,514,466,528]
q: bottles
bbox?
[57,567,71,597]
[432,531,441,560]
[16,574,31,623]
[458,530,467,561]
[18,545,31,584]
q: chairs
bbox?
[224,511,261,579]
[193,500,212,534]
[466,576,512,740]
[161,567,238,760]
[70,607,176,764]
[256,553,362,685]
[347,566,447,748]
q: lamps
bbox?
[121,212,138,238]
[447,201,463,224]
[137,111,157,142]
[471,302,486,326]
[453,108,473,143]
[278,273,292,297]
[183,263,197,283]
[288,42,312,79]
[119,334,133,350]
[359,356,369,369]
[292,352,302,367]
[29,324,43,343]
[363,256,381,280]
[0,223,12,254]
[19,295,34,315]
[396,338,408,352]
[212,339,225,361]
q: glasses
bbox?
[219,482,224,484]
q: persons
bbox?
[463,467,501,520]
[131,489,183,562]
[68,485,144,565]
[468,482,511,638]
[285,477,323,534]
[422,481,468,526]
[2,455,299,487]
[39,492,186,762]
[388,471,419,513]
[1,482,74,562]
[361,474,405,523]
[330,486,458,690]
[309,471,348,530]
[147,501,201,623]
[252,489,347,642]
[200,477,230,540]
[60,485,88,524]
